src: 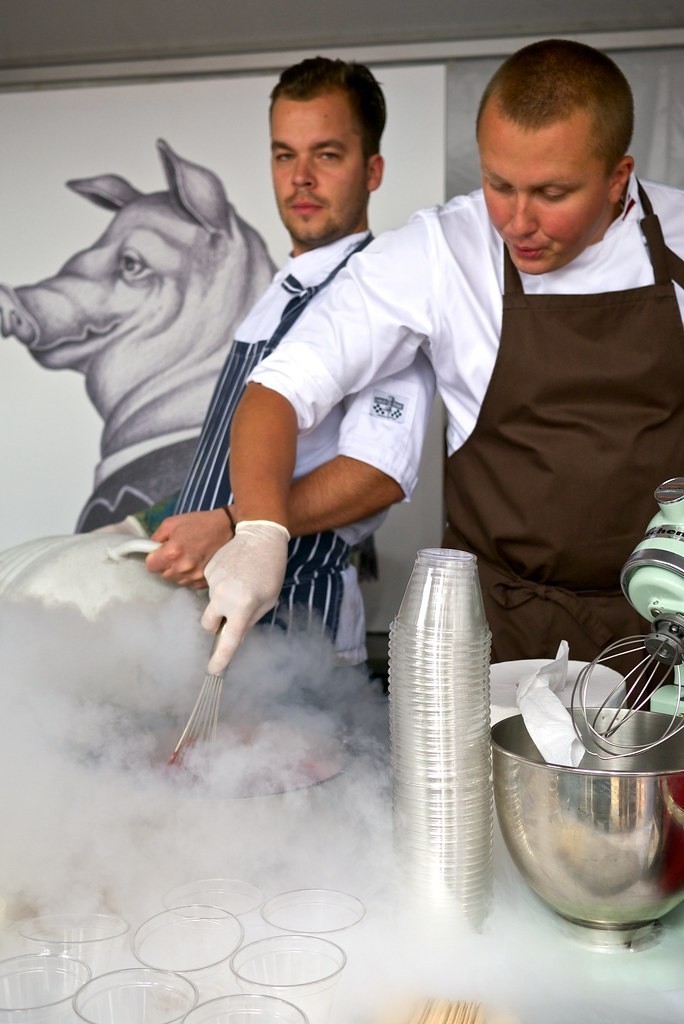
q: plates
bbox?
[489,655,630,782]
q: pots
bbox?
[157,698,355,810]
[1,652,393,938]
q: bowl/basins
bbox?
[490,706,683,945]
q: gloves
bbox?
[201,519,290,680]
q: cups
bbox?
[182,993,309,1024]
[229,933,346,1023]
[0,956,91,1023]
[259,890,367,944]
[71,968,199,1024]
[387,547,493,932]
[162,878,266,934]
[13,913,131,978]
[132,904,244,988]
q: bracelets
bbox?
[222,505,236,541]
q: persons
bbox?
[96,56,435,667]
[199,40,684,676]
